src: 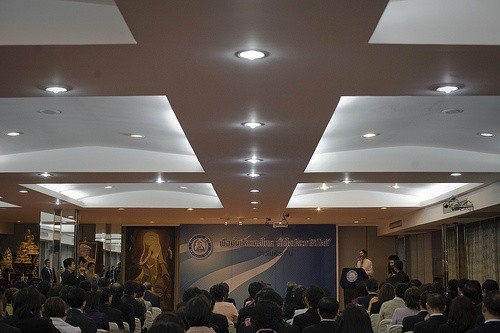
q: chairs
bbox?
[370,314,379,333]
[379,319,392,333]
[388,324,403,333]
[94,304,237,333]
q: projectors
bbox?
[273,222,288,228]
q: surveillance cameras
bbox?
[443,195,456,208]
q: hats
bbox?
[80,244,91,251]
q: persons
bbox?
[79,245,95,268]
[62,259,77,287]
[41,259,57,282]
[388,256,399,277]
[390,262,409,284]
[85,262,100,281]
[0,278,159,333]
[149,282,372,333]
[353,279,500,333]
[355,250,375,276]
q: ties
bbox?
[360,261,364,268]
[49,268,53,277]
